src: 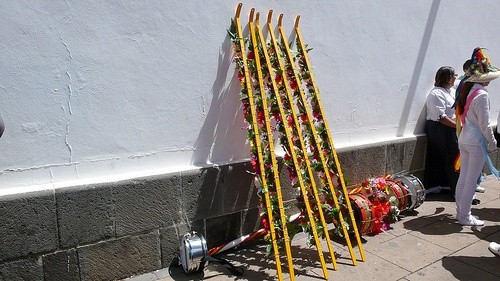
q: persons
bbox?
[488,242,500,257]
[455,47,500,226]
[456,60,485,193]
[427,67,480,205]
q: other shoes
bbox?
[488,241,500,255]
[472,197,480,206]
[455,212,485,226]
[476,185,486,193]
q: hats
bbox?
[461,47,500,82]
